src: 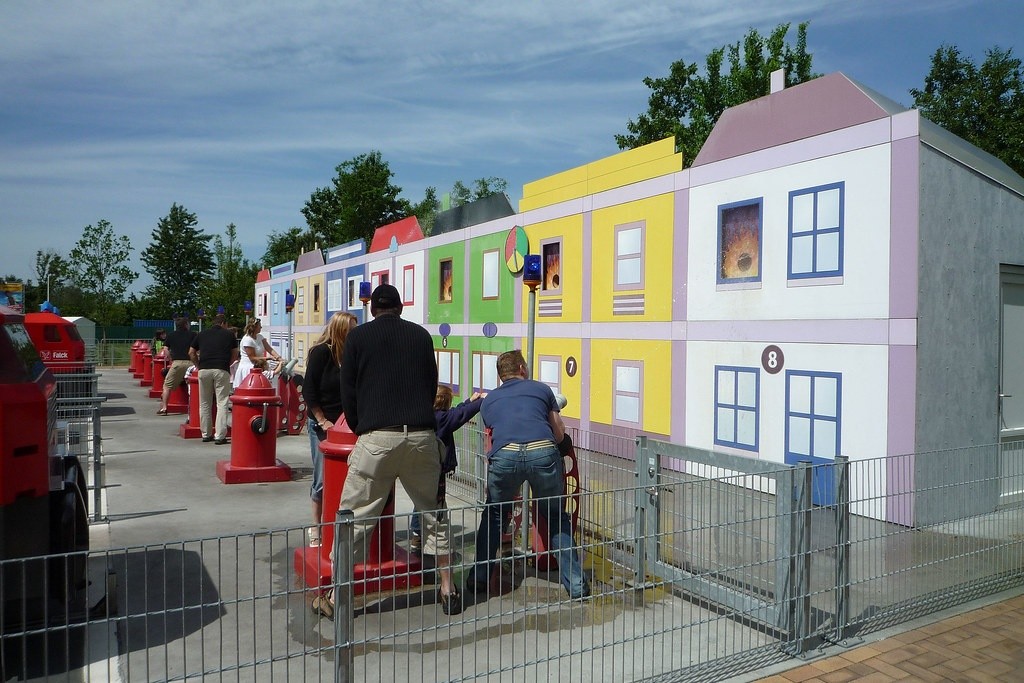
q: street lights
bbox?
[47,273,52,302]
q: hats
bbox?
[371,284,401,309]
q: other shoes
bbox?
[468,576,487,593]
[439,583,462,615]
[185,419,190,424]
[215,438,227,445]
[410,533,422,548]
[307,526,323,547]
[312,589,334,621]
[571,586,592,602]
[202,436,213,442]
[157,409,168,416]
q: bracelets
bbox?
[318,419,327,426]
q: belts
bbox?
[369,424,435,431]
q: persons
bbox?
[466,351,590,601]
[155,317,198,416]
[301,285,591,622]
[149,315,287,445]
[312,285,462,621]
